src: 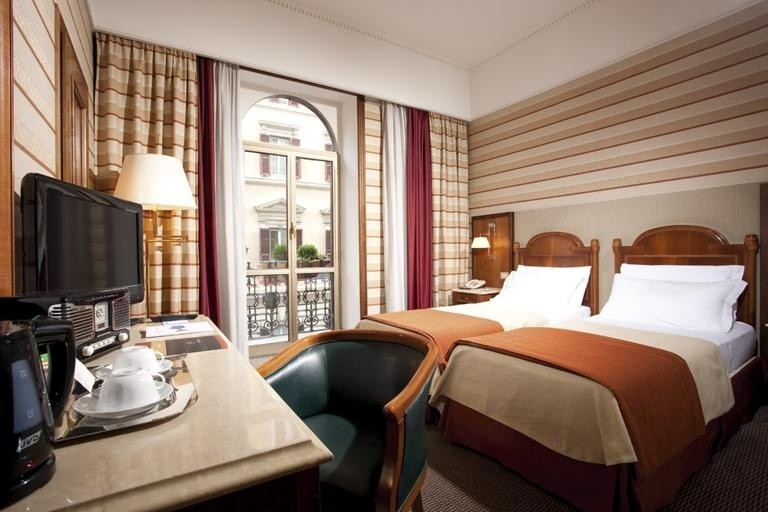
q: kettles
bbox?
[2,317,77,511]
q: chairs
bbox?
[255,328,439,512]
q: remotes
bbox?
[150,313,198,321]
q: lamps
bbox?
[114,154,199,324]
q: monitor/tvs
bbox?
[22,173,145,325]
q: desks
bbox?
[0,315,335,511]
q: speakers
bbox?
[49,287,130,361]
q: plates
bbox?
[98,358,174,379]
[71,380,176,421]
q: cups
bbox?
[94,368,167,411]
[112,345,165,371]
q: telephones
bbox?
[463,279,486,289]
